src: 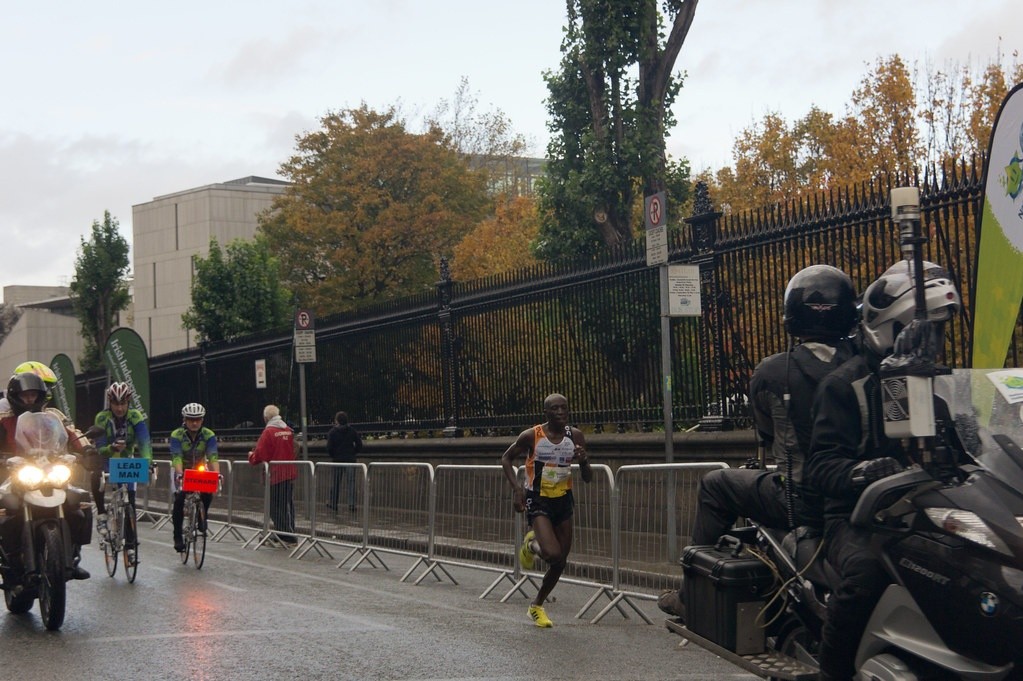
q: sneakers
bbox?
[96,514,108,535]
[520,531,538,570]
[125,548,142,565]
[526,602,553,628]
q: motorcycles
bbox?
[0,410,106,631]
[664,370,1022,681]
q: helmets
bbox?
[5,372,51,417]
[779,264,861,346]
[11,360,60,403]
[106,381,130,404]
[859,258,962,358]
[180,402,206,419]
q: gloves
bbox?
[83,445,103,470]
[848,455,904,496]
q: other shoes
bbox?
[265,535,299,547]
[349,505,357,511]
[71,565,91,581]
[173,533,184,551]
[198,517,208,533]
[326,503,338,511]
[657,587,687,621]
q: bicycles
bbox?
[93,462,160,586]
[174,466,223,570]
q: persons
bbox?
[90,381,159,565]
[326,412,363,512]
[501,393,593,628]
[248,405,300,548]
[0,360,100,591]
[657,259,981,681]
[170,403,224,551]
